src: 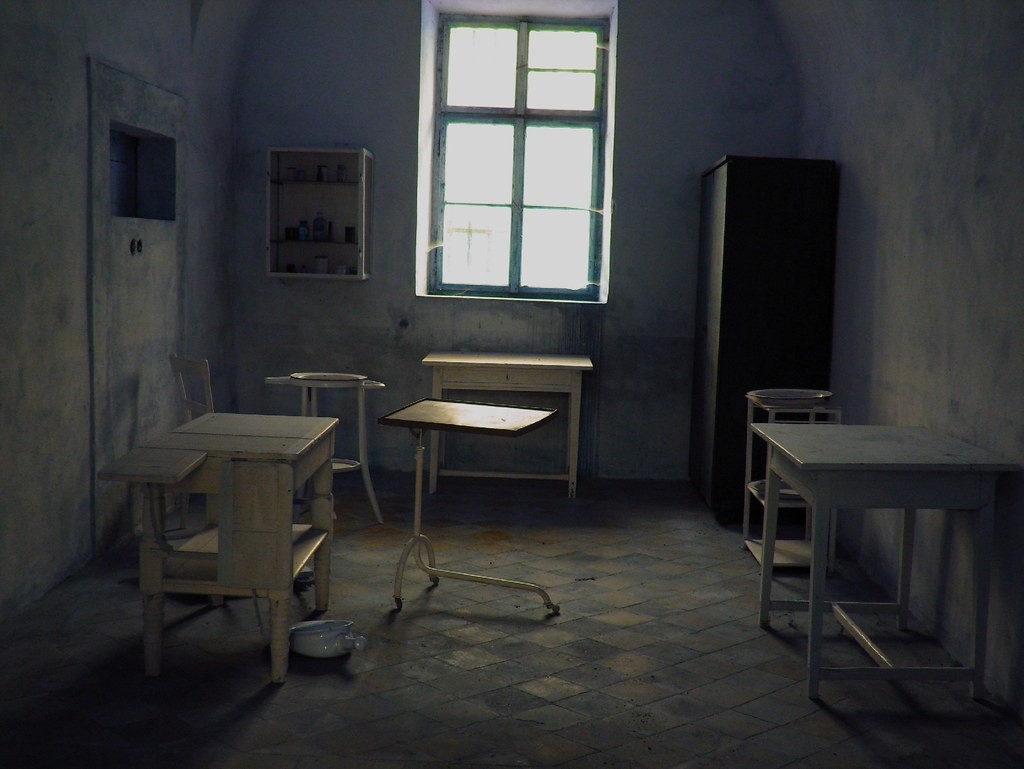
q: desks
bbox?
[98,412,340,683]
[378,398,560,618]
[749,422,1019,702]
[422,350,592,498]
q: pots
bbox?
[291,619,367,657]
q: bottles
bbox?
[312,212,327,240]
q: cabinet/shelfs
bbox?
[741,395,840,577]
[691,153,844,526]
[262,371,384,528]
[266,145,372,281]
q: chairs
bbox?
[171,353,217,528]
